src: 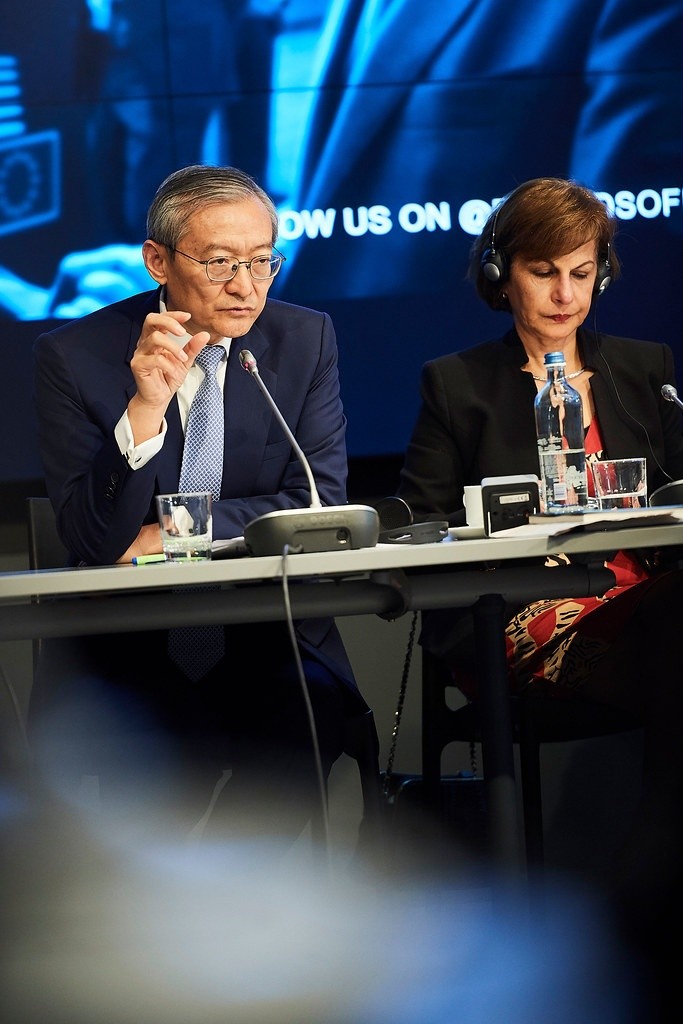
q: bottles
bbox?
[534,351,588,516]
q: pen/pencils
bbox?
[132,554,165,565]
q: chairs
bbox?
[419,611,683,899]
[25,497,386,885]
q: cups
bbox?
[155,491,212,562]
[591,458,648,512]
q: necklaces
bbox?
[529,368,587,384]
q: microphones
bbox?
[661,384,683,410]
[238,350,379,555]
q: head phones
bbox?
[346,497,449,544]
[481,185,612,294]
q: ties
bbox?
[179,345,223,508]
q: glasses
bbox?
[164,242,287,281]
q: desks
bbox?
[0,504,683,642]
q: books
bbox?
[525,504,683,535]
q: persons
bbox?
[391,174,682,833]
[26,162,389,873]
